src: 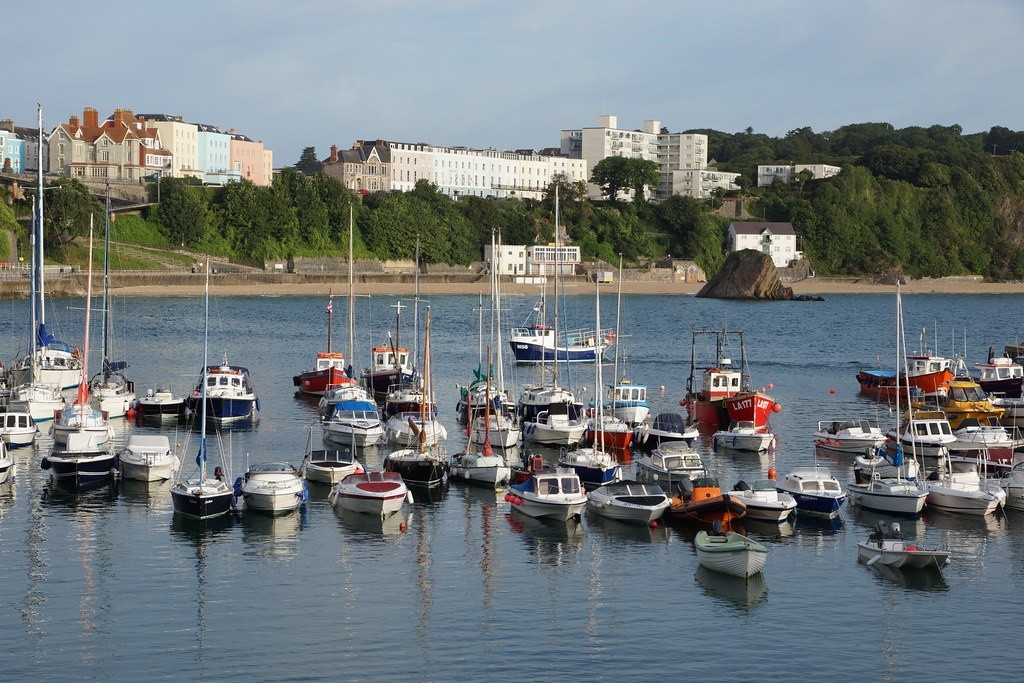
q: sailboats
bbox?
[49,211,109,445]
[89,177,136,420]
[0,104,83,424]
[384,305,451,490]
[169,254,236,518]
[847,277,930,514]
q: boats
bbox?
[241,452,308,514]
[119,436,180,483]
[360,301,424,395]
[0,378,42,449]
[299,398,365,486]
[506,317,1024,578]
[137,385,184,419]
[292,182,651,486]
[40,452,118,488]
[383,408,448,448]
[329,468,410,515]
[185,346,262,424]
[0,435,16,484]
